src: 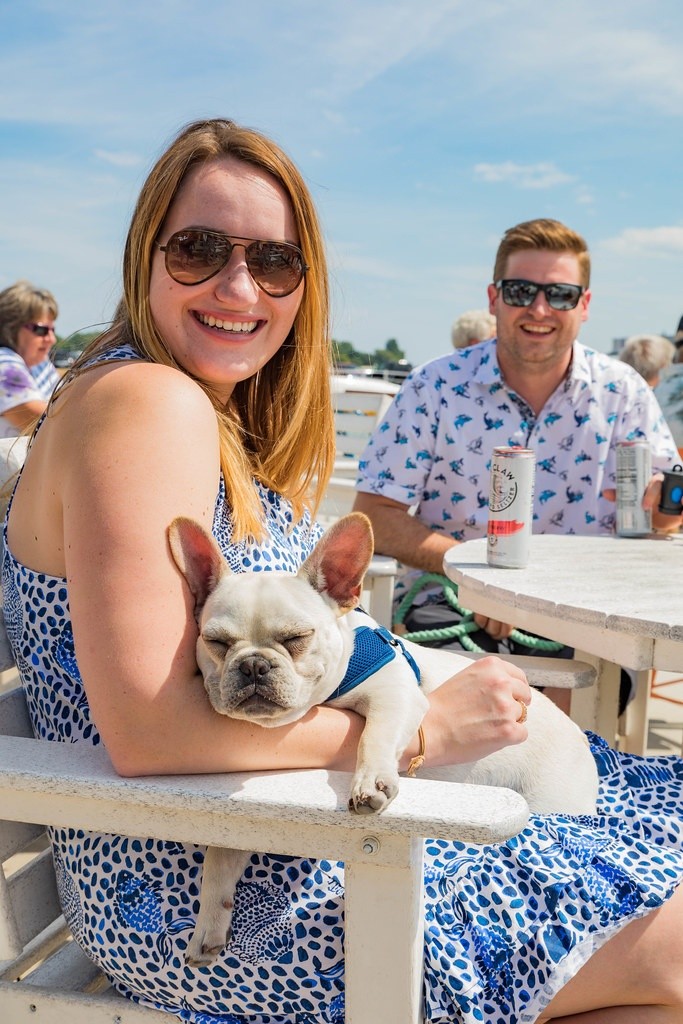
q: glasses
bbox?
[23,322,54,336]
[496,279,585,311]
[155,229,310,298]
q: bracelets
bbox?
[406,725,425,785]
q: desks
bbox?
[441,532,683,758]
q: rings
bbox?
[516,696,530,724]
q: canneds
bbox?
[487,446,535,569]
[614,440,653,537]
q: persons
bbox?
[618,312,682,453]
[355,215,683,730]
[0,282,76,529]
[448,308,498,353]
[0,115,682,1024]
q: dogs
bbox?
[166,511,600,969]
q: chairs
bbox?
[0,494,599,1024]
[295,390,406,523]
[360,548,650,756]
